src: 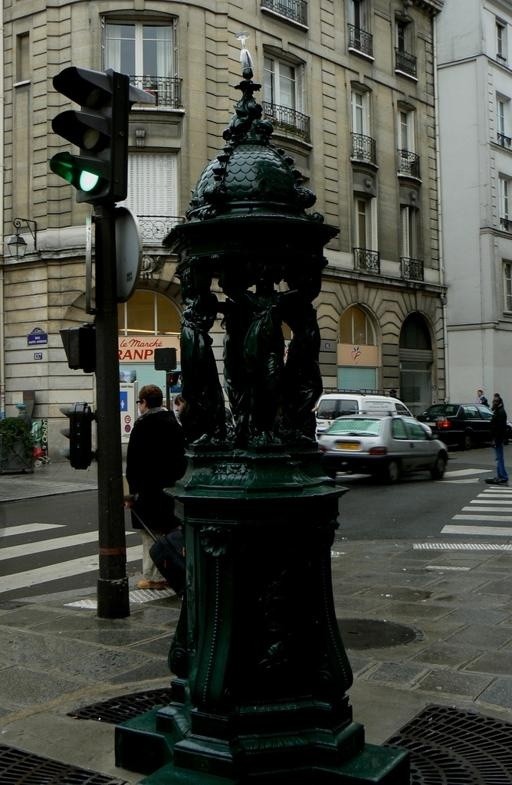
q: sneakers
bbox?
[137,580,168,589]
[494,477,508,482]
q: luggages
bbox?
[130,507,185,592]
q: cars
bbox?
[317,414,449,484]
[417,402,512,449]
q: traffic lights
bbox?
[58,401,93,471]
[47,66,157,204]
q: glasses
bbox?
[136,401,142,406]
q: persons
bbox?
[174,393,188,415]
[491,393,500,406]
[387,389,397,398]
[488,392,509,484]
[476,390,484,404]
[125,384,189,591]
[479,396,489,407]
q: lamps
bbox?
[7,218,36,260]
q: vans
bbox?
[313,392,414,438]
